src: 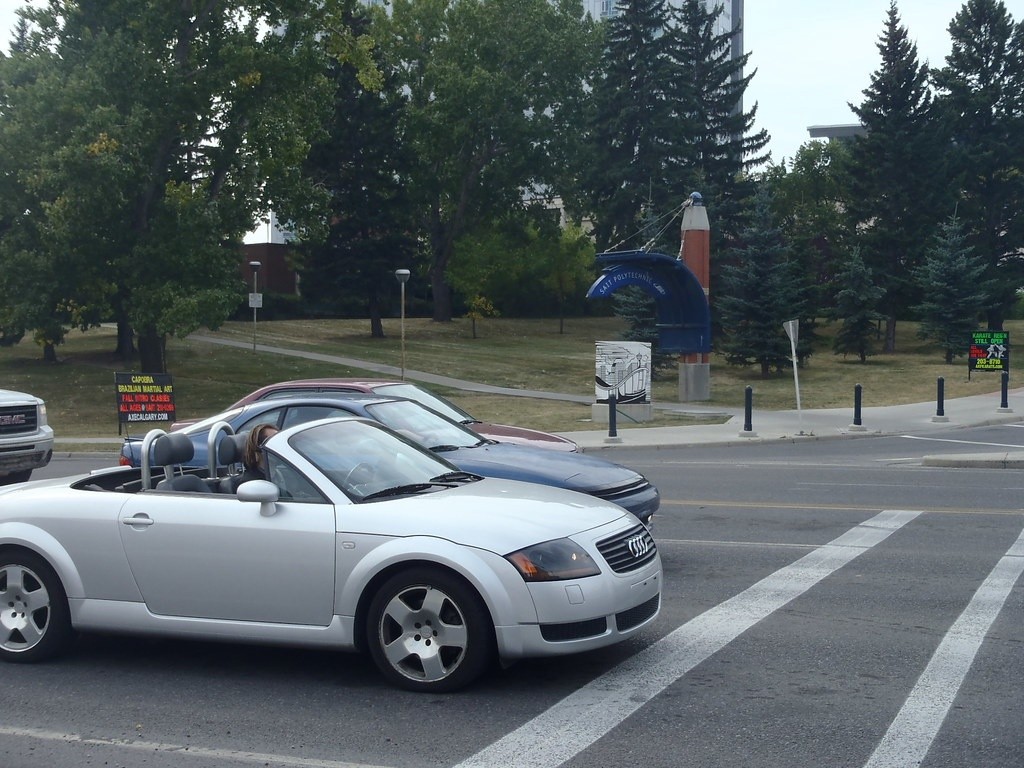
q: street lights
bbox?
[394,267,408,383]
[246,259,262,353]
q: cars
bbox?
[0,379,57,481]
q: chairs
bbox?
[154,432,214,493]
[217,431,248,494]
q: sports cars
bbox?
[0,402,664,696]
[120,392,660,544]
[168,378,580,457]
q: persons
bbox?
[229,423,292,502]
